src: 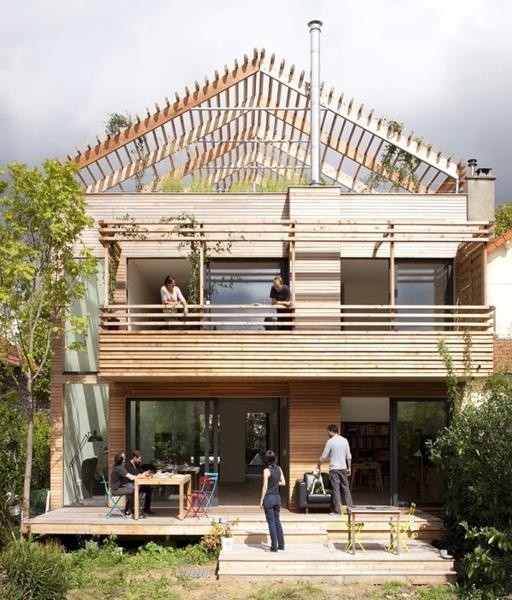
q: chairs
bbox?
[338,503,366,553]
[297,473,335,514]
[125,492,145,513]
[386,502,417,554]
[191,471,218,515]
[100,474,127,517]
[183,475,209,520]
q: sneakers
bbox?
[265,545,285,552]
[124,510,156,517]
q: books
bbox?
[341,423,390,461]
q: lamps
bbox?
[68,430,103,505]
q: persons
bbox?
[158,275,190,329]
[109,451,146,519]
[259,450,286,552]
[268,276,294,330]
[124,451,156,516]
[317,424,355,516]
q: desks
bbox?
[347,505,400,554]
[133,474,192,520]
[349,462,384,493]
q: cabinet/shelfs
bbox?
[341,421,403,490]
